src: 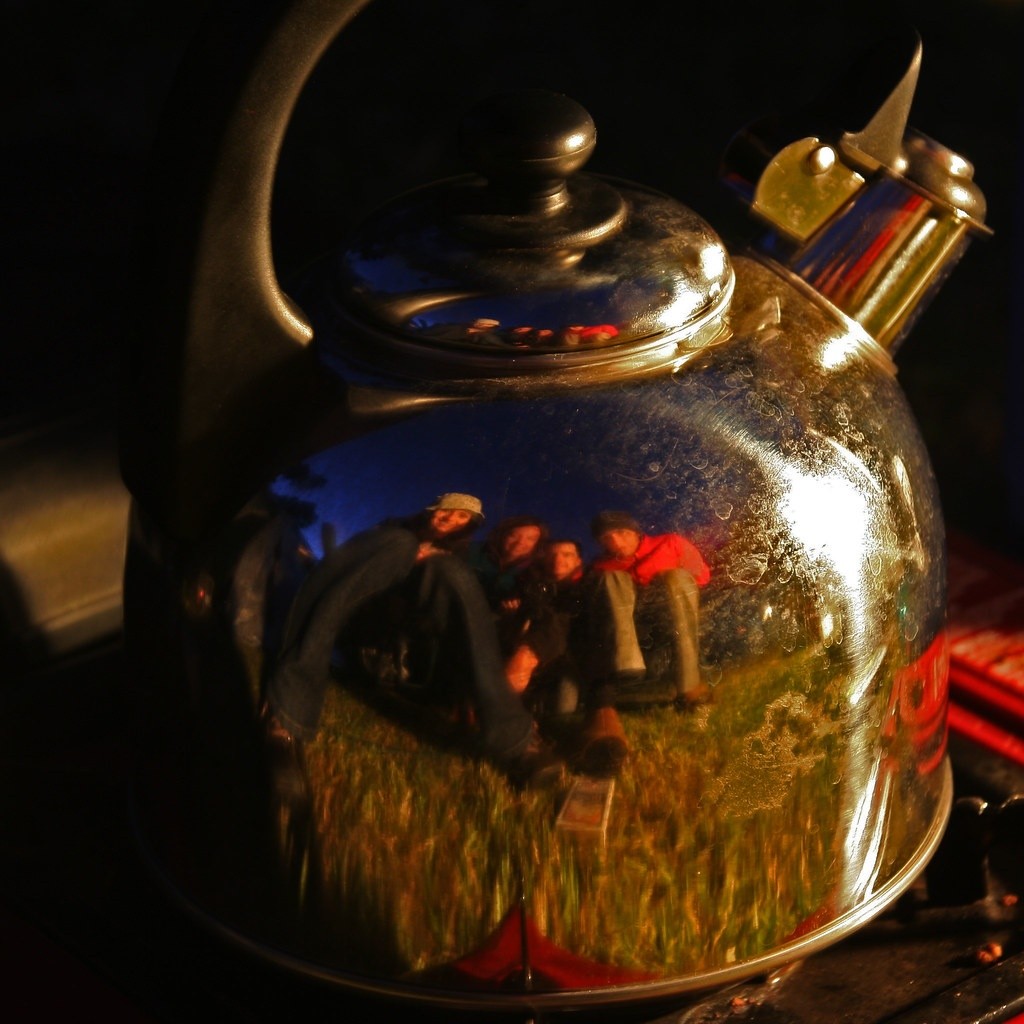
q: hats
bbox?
[424,493,485,521]
[591,510,642,540]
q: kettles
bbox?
[118,0,992,1011]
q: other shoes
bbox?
[509,733,569,787]
[677,682,713,712]
[261,715,295,743]
[583,707,629,779]
[617,673,646,693]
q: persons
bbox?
[298,494,711,778]
